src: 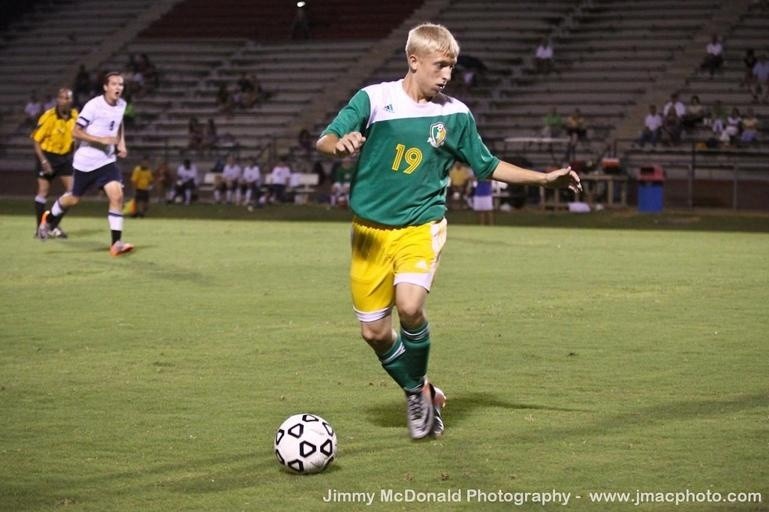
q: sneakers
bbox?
[48,228,66,239]
[405,375,434,439]
[430,383,447,438]
[38,211,49,241]
[111,239,132,256]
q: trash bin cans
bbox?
[636,164,666,212]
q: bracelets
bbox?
[40,159,49,165]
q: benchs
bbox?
[0,1,769,219]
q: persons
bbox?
[28,85,80,241]
[580,126,605,143]
[541,105,562,138]
[38,69,136,257]
[447,52,489,92]
[533,36,556,74]
[23,46,159,130]
[561,124,587,151]
[128,156,154,217]
[634,33,769,151]
[565,107,583,128]
[151,70,357,212]
[443,156,573,226]
[312,20,583,442]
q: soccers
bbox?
[275,413,335,471]
